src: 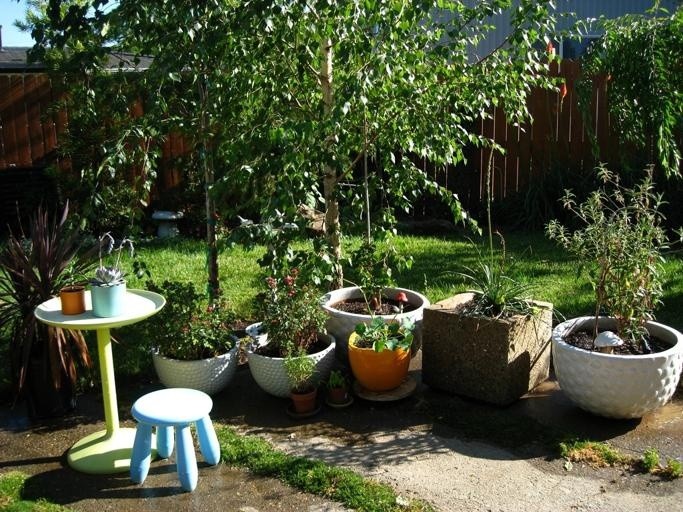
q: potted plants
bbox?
[88,231,136,318]
[316,224,429,367]
[422,143,552,407]
[544,160,682,418]
[324,370,354,407]
[137,278,242,400]
[229,216,330,334]
[282,340,322,416]
[53,256,88,315]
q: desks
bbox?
[34,286,168,474]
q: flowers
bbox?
[247,268,328,351]
[347,291,418,352]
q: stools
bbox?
[130,388,221,493]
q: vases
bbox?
[243,330,333,396]
[344,329,413,392]
